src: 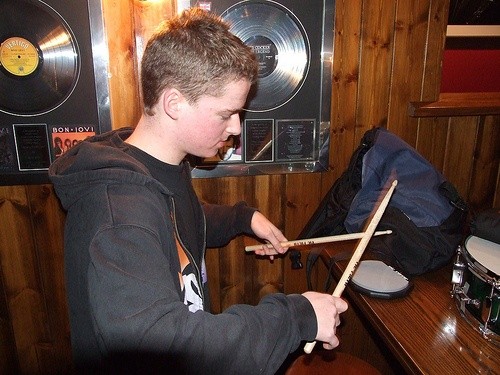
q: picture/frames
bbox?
[0,0,112,186]
[184,0,336,180]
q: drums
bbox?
[451,234,500,345]
[350,259,414,298]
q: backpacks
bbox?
[283,127,469,287]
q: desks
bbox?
[310,235,500,375]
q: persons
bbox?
[203,136,241,161]
[46,7,382,374]
